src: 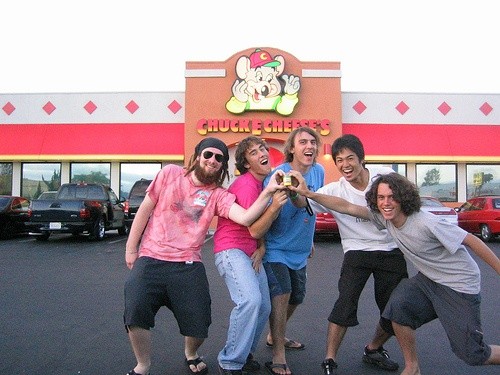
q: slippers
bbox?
[127,363,150,375]
[185,356,208,375]
[266,338,306,350]
[264,362,293,375]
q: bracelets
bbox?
[290,192,299,200]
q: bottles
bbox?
[283,175,291,186]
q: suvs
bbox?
[122,177,154,228]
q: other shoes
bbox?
[242,353,261,372]
[216,364,242,375]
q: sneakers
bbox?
[320,358,338,375]
[362,344,399,370]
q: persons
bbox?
[289,172,500,375]
[250,126,325,375]
[122,137,286,375]
[213,136,289,375]
[288,134,409,375]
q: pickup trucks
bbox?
[25,183,129,242]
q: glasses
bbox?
[202,151,224,162]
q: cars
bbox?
[417,194,458,226]
[37,191,59,201]
[453,194,499,240]
[314,212,342,233]
[0,195,32,239]
